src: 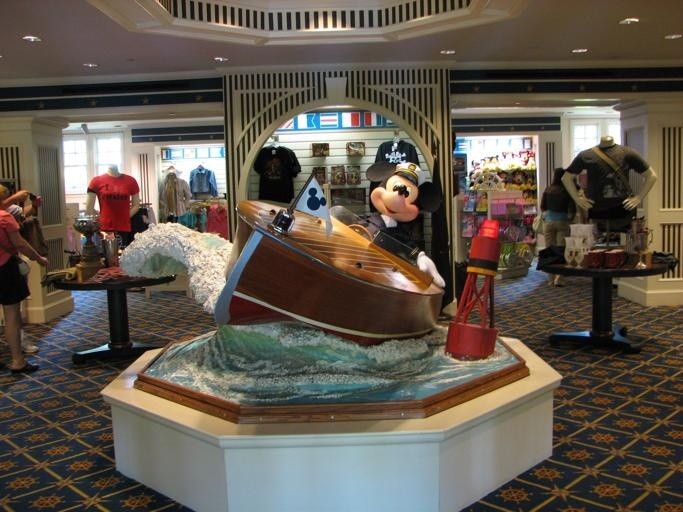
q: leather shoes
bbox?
[554,281,566,285]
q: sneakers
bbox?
[22,343,39,354]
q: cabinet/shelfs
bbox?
[465,189,528,280]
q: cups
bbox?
[101,238,118,268]
[584,248,627,268]
[569,224,593,247]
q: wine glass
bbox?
[563,237,574,267]
[631,232,648,269]
[574,238,584,269]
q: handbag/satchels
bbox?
[19,263,32,275]
[532,215,545,234]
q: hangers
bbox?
[162,161,206,174]
[387,129,402,146]
[264,134,282,148]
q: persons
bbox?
[84,164,140,250]
[0,180,49,373]
[540,168,577,286]
[561,135,657,245]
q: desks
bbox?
[53,265,176,365]
[540,253,676,353]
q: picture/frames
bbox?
[331,165,345,185]
[310,142,330,158]
[345,141,365,157]
[347,166,361,185]
[329,188,366,209]
[312,166,326,184]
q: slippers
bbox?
[10,361,39,373]
